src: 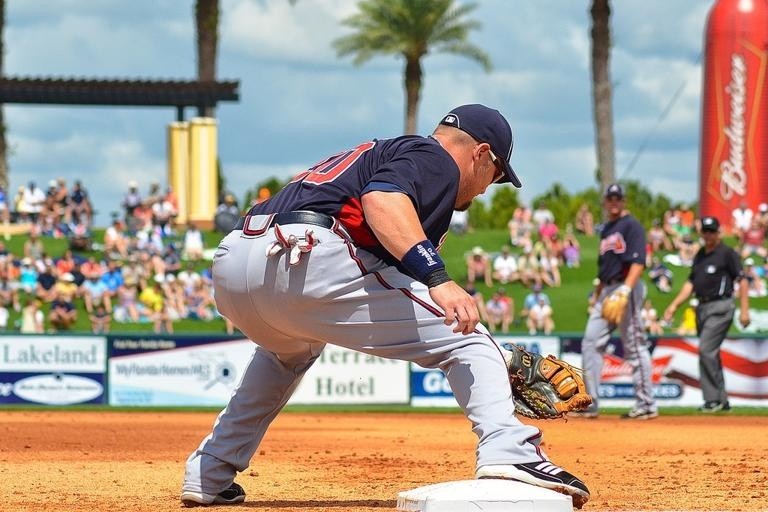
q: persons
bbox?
[662,216,750,412]
[448,200,768,335]
[566,184,658,419]
[1,178,272,335]
[182,104,589,506]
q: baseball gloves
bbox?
[601,285,631,324]
[502,343,592,419]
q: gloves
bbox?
[264,222,318,265]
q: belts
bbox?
[599,277,627,285]
[697,293,734,303]
[232,210,337,233]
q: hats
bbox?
[699,216,722,235]
[602,182,626,203]
[437,103,525,193]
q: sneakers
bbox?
[620,402,659,420]
[474,459,591,506]
[697,399,732,415]
[179,481,247,506]
[566,405,599,420]
